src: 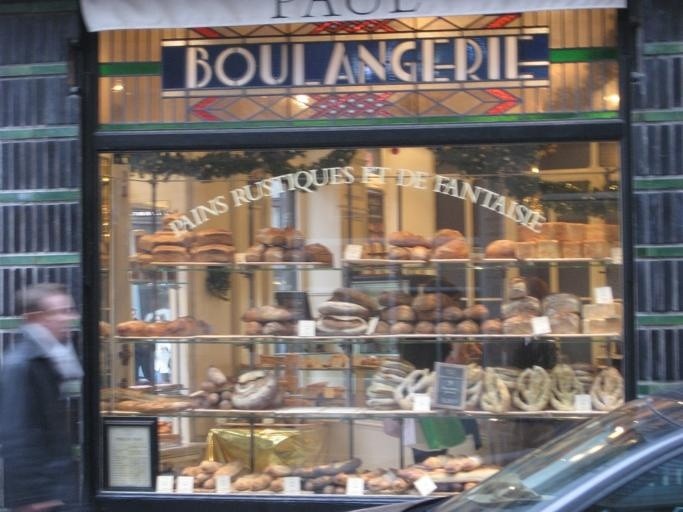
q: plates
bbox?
[252,331,275,341]
[103,406,183,416]
[540,332,622,337]
[430,258,467,263]
[236,261,323,267]
[523,258,594,262]
[343,258,424,266]
[195,334,254,340]
[481,258,518,263]
[276,332,361,342]
[277,409,454,420]
[360,333,444,337]
[454,408,606,418]
[443,333,538,338]
[185,408,276,419]
[151,260,227,266]
[114,334,191,339]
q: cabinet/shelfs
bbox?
[112,257,625,421]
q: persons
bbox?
[503,275,560,463]
[393,276,484,466]
[152,313,176,383]
[131,307,154,384]
[1,284,89,512]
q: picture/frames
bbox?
[431,362,467,411]
[98,417,159,492]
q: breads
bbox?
[138,213,235,265]
[260,348,395,407]
[116,315,210,336]
[314,288,501,335]
[100,382,195,414]
[388,229,470,261]
[244,228,333,263]
[187,364,274,409]
[180,453,504,494]
[240,306,296,335]
[501,275,624,332]
[487,221,621,259]
